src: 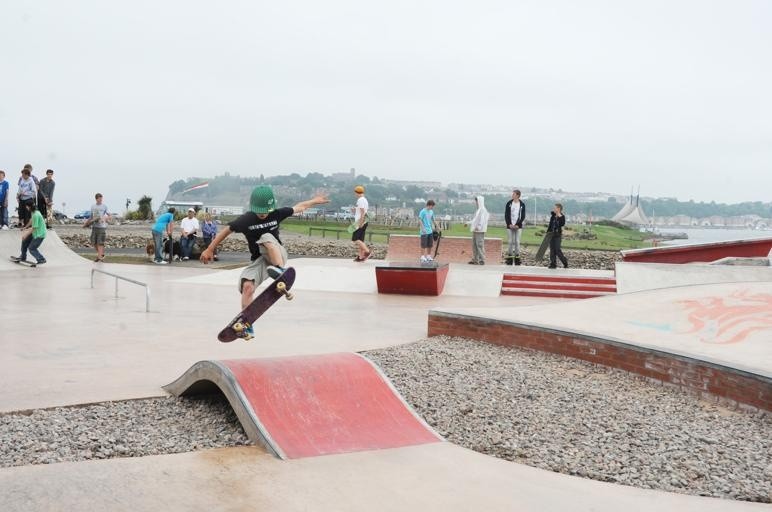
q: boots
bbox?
[549,259,556,269]
[515,254,521,265]
[505,255,513,265]
[560,256,568,268]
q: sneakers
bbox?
[353,251,371,262]
[2,222,23,230]
[153,257,167,264]
[420,257,434,262]
[266,264,285,280]
[468,261,485,265]
[183,256,190,260]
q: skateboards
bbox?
[347,212,375,233]
[10,256,46,267]
[536,232,553,261]
[433,231,443,260]
[218,267,295,343]
[169,235,173,263]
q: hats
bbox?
[249,186,275,214]
[188,208,194,212]
[354,186,364,193]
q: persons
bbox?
[151,207,218,266]
[468,195,489,264]
[352,186,371,262]
[418,200,438,262]
[198,186,332,338]
[0,164,56,265]
[86,193,112,262]
[546,204,568,268]
[505,191,525,265]
